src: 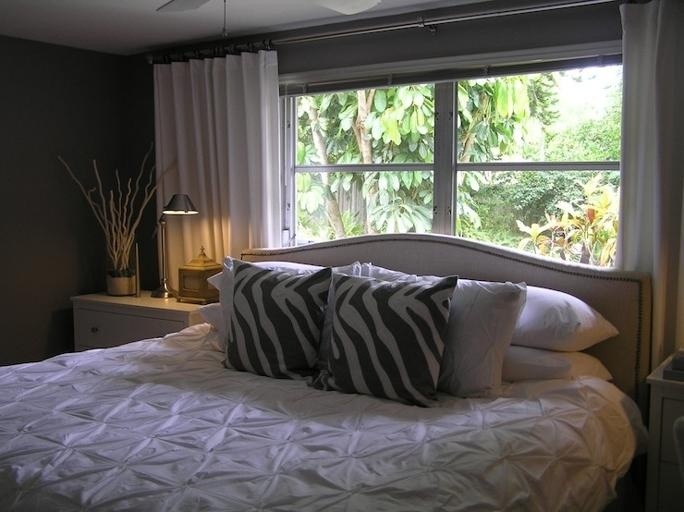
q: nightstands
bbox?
[644,348,684,512]
[70,291,204,352]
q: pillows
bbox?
[205,261,326,293]
[369,264,526,397]
[503,345,614,383]
[221,259,334,385]
[221,256,360,350]
[312,274,459,408]
[511,285,618,352]
[200,303,224,333]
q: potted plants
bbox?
[57,142,156,296]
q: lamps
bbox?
[150,193,200,298]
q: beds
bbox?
[0,233,652,512]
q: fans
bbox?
[156,1,381,39]
